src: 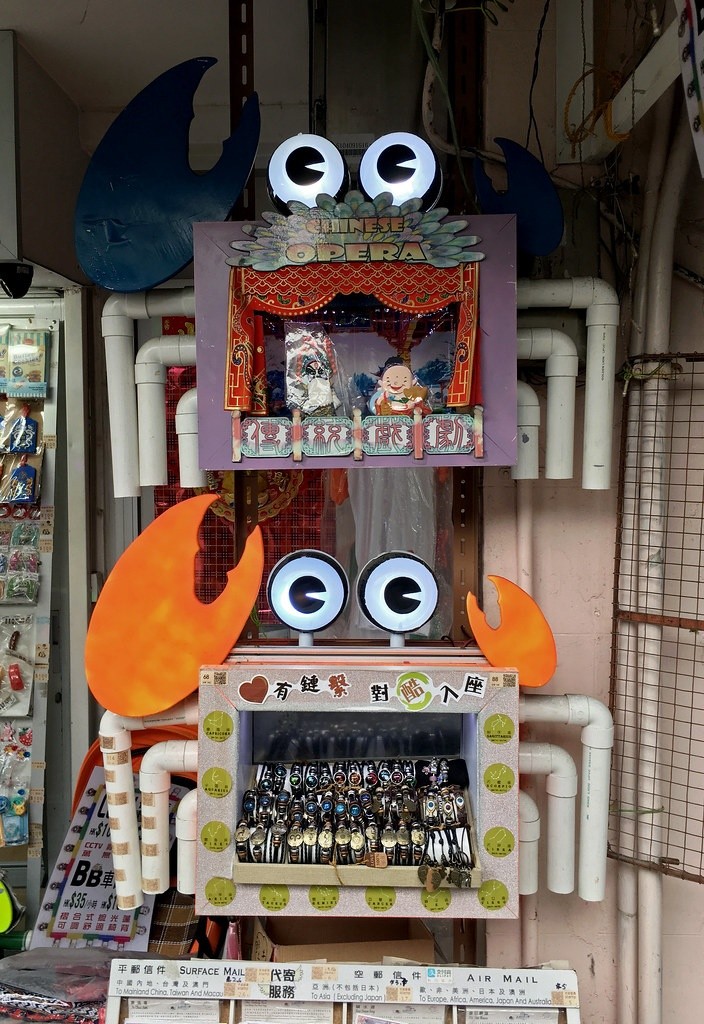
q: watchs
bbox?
[234,757,469,866]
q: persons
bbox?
[285,322,342,419]
[369,356,432,417]
